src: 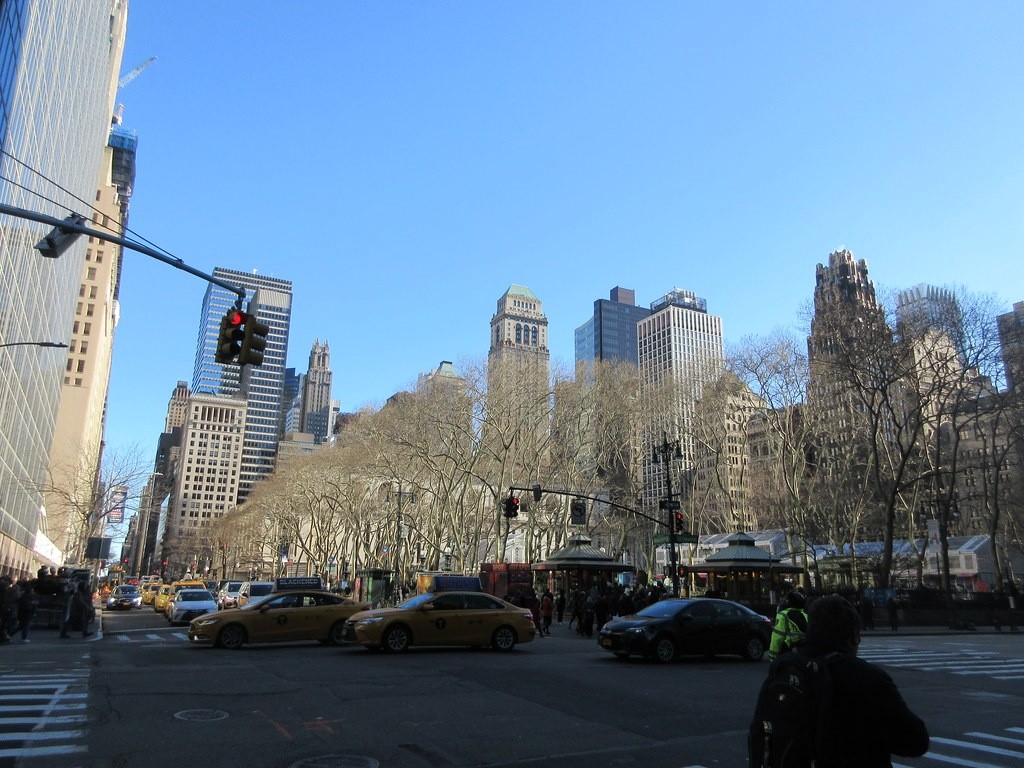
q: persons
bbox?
[849,594,874,631]
[886,597,898,631]
[568,591,608,638]
[805,593,929,768]
[60,582,94,638]
[798,587,817,597]
[511,590,529,608]
[992,609,1002,631]
[527,593,544,638]
[705,586,716,598]
[0,575,33,643]
[556,593,566,625]
[541,589,553,634]
[768,591,808,664]
[591,582,669,617]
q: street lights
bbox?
[384,481,416,606]
[91,472,164,593]
[651,432,683,597]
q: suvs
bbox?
[123,575,140,585]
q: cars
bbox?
[106,585,143,609]
[598,598,773,664]
[340,590,536,653]
[188,590,373,649]
[200,580,275,609]
[24,576,78,629]
[139,580,219,626]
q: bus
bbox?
[106,565,122,585]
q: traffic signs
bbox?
[659,500,681,510]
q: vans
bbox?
[141,575,164,583]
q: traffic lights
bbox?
[239,314,269,366]
[124,555,128,562]
[675,512,683,530]
[678,566,687,575]
[509,497,519,517]
[218,309,246,360]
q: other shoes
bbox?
[60,635,71,639]
[543,628,545,632]
[547,632,550,634]
[84,631,93,637]
[6,634,13,643]
[21,638,32,643]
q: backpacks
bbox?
[749,645,857,768]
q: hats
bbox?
[784,591,806,609]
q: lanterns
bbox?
[152,568,159,573]
[191,564,198,568]
[161,560,168,566]
[123,557,129,563]
